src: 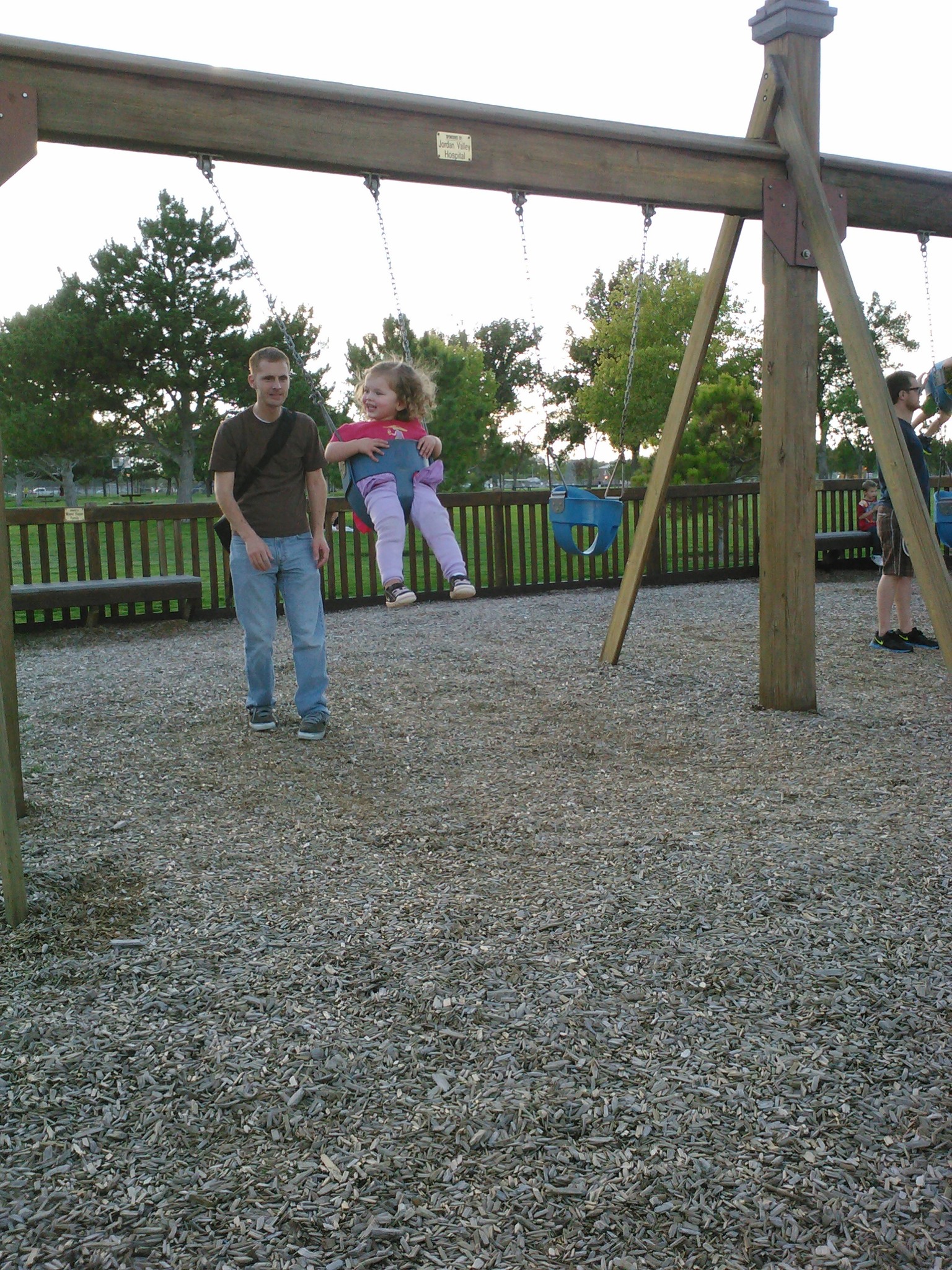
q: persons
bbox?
[323,351,476,607]
[910,356,952,456]
[207,346,332,740]
[867,370,940,654]
[856,480,885,567]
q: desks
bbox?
[514,487,540,491]
[36,495,54,505]
[121,494,141,501]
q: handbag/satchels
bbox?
[214,513,232,553]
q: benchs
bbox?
[756,530,872,566]
[109,501,154,505]
[10,575,203,633]
[39,500,60,502]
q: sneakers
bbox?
[384,584,417,607]
[449,576,476,599]
[869,627,914,652]
[248,706,276,730]
[298,709,327,741]
[896,627,939,649]
[870,554,885,566]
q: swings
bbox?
[504,187,657,559]
[914,229,952,548]
[187,152,440,528]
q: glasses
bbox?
[897,387,922,397]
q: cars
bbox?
[28,487,47,494]
